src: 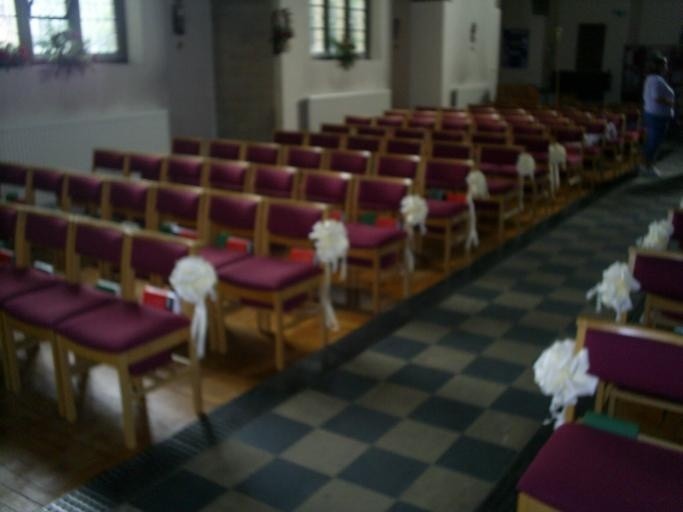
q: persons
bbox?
[640,49,677,177]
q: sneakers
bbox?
[638,165,660,178]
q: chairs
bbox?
[91,148,127,175]
[512,309,682,512]
[301,169,354,308]
[1,206,22,271]
[217,197,340,368]
[66,173,103,215]
[6,218,125,418]
[475,143,525,235]
[104,179,152,227]
[128,154,164,182]
[592,244,682,418]
[344,174,413,306]
[420,160,477,265]
[55,233,204,451]
[190,191,261,352]
[154,183,204,239]
[173,103,641,203]
[33,167,64,208]
[1,162,31,205]
[645,204,682,325]
[3,209,70,391]
